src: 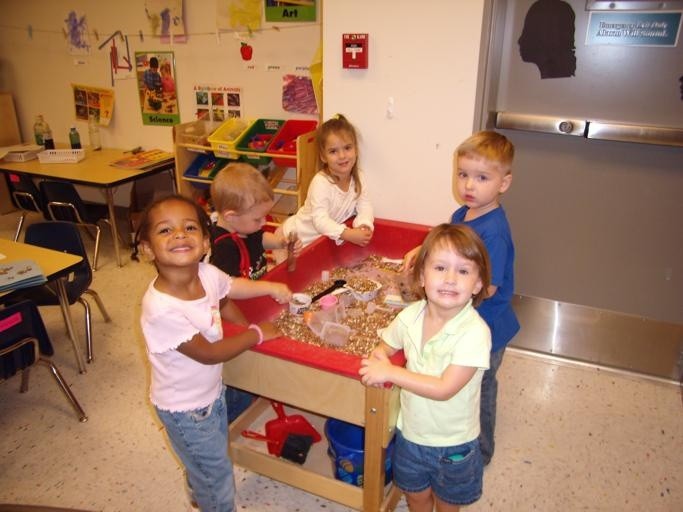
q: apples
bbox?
[240,41,253,61]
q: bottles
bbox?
[42,122,55,149]
[285,218,299,272]
[87,114,102,150]
[67,124,81,149]
[32,111,46,145]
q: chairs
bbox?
[39,180,124,271]
[22,220,112,361]
[0,298,89,424]
[4,171,41,243]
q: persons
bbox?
[130,194,293,511]
[143,56,162,97]
[358,223,493,512]
[273,113,376,267]
[160,63,176,99]
[399,131,522,469]
[201,162,303,426]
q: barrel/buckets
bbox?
[323,417,397,489]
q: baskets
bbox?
[3,146,45,163]
[37,148,88,164]
[180,118,321,192]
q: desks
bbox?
[0,140,176,270]
[0,239,88,375]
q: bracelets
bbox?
[248,323,264,344]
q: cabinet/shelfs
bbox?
[173,120,321,238]
[218,216,441,512]
[0,89,28,214]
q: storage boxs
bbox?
[265,185,298,225]
[236,119,286,164]
[267,119,318,166]
[207,116,256,160]
[183,153,223,188]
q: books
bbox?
[108,149,180,170]
[0,259,48,290]
